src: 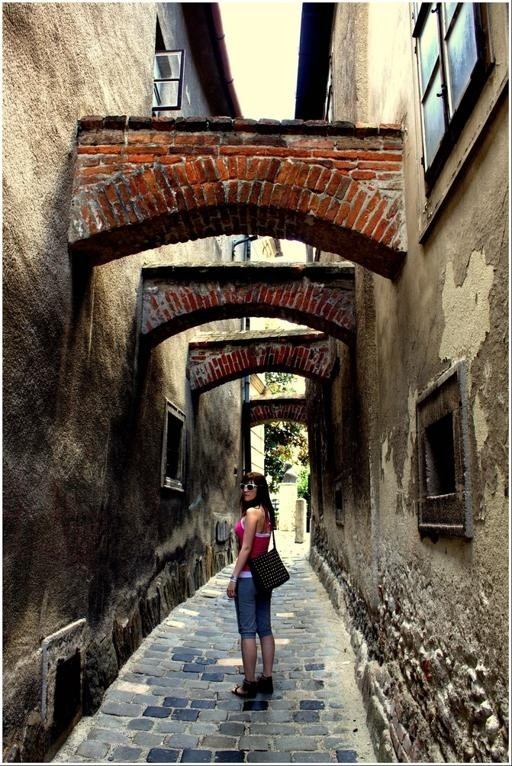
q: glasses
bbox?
[239,483,259,490]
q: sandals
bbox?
[257,674,274,695]
[231,680,257,698]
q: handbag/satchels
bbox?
[246,548,290,593]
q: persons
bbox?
[226,471,275,699]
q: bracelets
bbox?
[229,574,239,583]
